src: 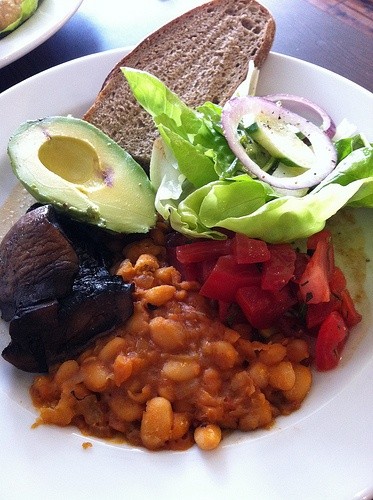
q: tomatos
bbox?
[173,233,362,373]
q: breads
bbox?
[79,0,276,175]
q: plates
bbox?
[1,45,373,500]
[0,1,83,69]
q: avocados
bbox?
[5,116,160,236]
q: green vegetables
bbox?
[118,57,373,243]
